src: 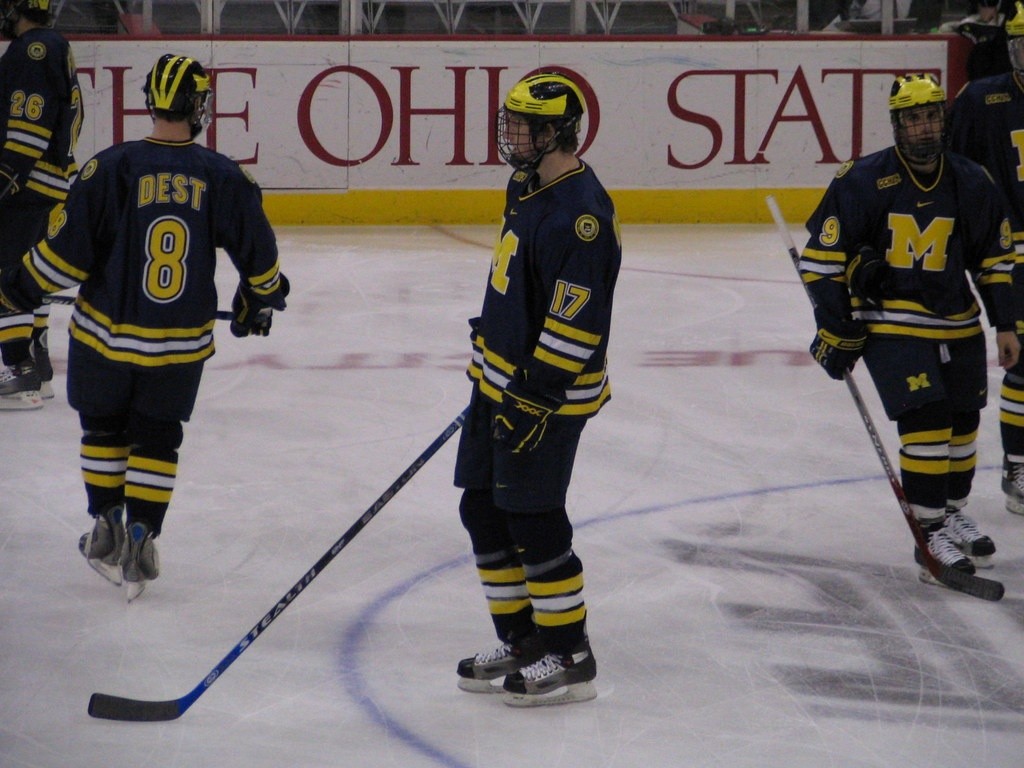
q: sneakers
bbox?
[79,501,125,586]
[454,627,547,692]
[121,515,160,602]
[31,326,55,398]
[1000,454,1024,516]
[915,521,976,594]
[502,610,597,709]
[944,507,997,569]
[0,338,45,410]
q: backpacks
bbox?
[1,164,27,200]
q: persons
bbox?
[451,71,626,697]
[939,0,1024,504]
[797,70,1022,577]
[0,0,87,401]
[0,54,291,582]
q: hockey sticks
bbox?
[48,296,273,327]
[765,193,1007,602]
[87,405,468,723]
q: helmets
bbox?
[496,74,586,171]
[0,0,50,40]
[144,53,217,140]
[1005,0,1024,71]
[888,72,954,160]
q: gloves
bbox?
[490,364,568,453]
[229,272,291,337]
[810,305,867,381]
[0,264,43,313]
